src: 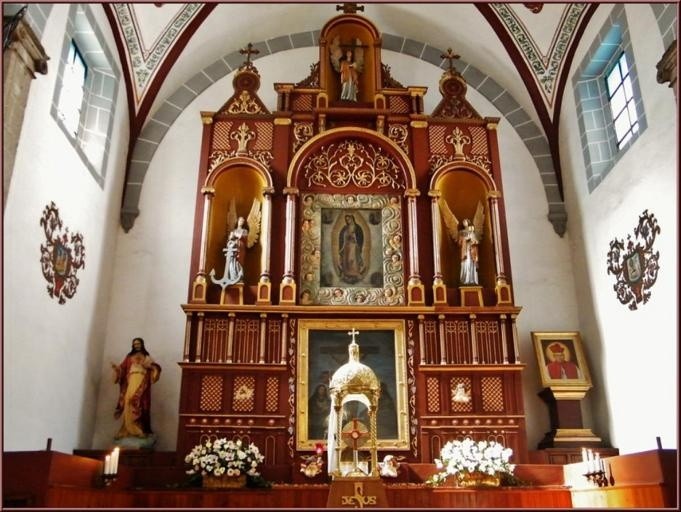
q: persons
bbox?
[339,48,362,103]
[110,335,162,442]
[387,254,402,267]
[308,384,331,440]
[299,289,313,305]
[355,294,367,303]
[332,288,344,298]
[345,195,356,204]
[226,215,248,270]
[303,195,315,204]
[456,217,482,284]
[339,213,367,281]
[375,381,399,440]
[387,232,402,251]
[544,343,585,380]
[388,196,400,205]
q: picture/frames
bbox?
[294,193,412,453]
[530,330,594,388]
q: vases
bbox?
[455,469,501,489]
[201,474,247,488]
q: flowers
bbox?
[425,439,517,484]
[183,439,265,479]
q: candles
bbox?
[581,447,604,474]
[103,447,119,475]
